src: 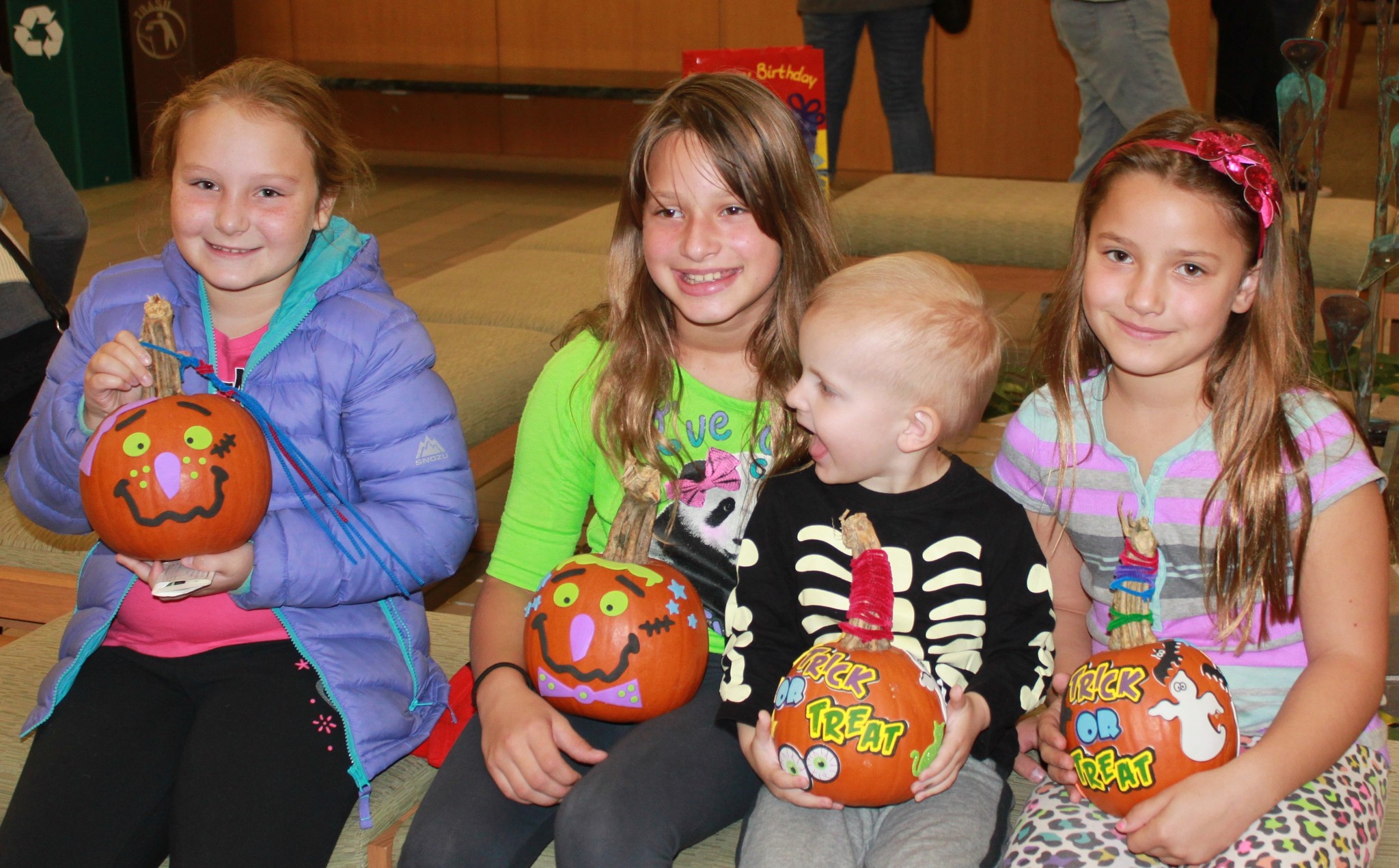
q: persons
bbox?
[797,0,936,183]
[1209,0,1320,157]
[718,250,1056,868]
[0,59,481,868]
[992,109,1389,867]
[1049,0,1195,183]
[398,72,842,868]
[0,64,87,456]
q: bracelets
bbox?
[472,662,532,708]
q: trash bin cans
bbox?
[127,1,233,180]
[3,0,133,192]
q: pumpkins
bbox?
[77,293,272,562]
[1060,520,1239,818]
[522,462,708,723]
[770,510,948,807]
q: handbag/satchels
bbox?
[0,314,76,466]
[682,45,828,196]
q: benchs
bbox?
[1,173,1399,868]
